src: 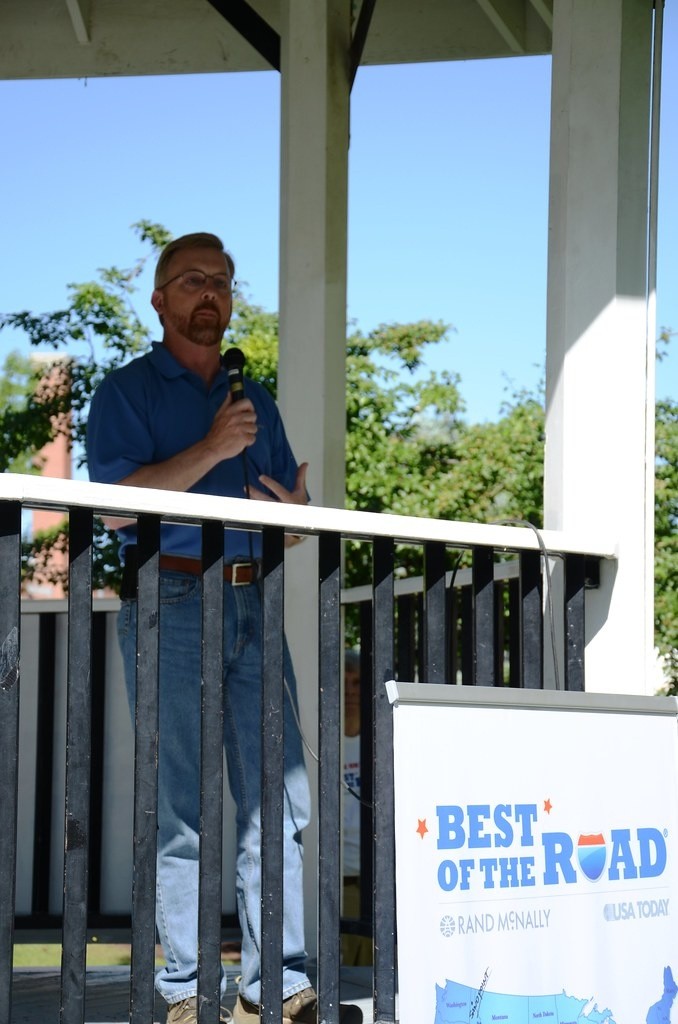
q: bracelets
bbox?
[292,536,304,540]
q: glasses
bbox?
[157,269,237,293]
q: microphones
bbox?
[223,346,247,406]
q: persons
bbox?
[339,648,373,966]
[84,233,367,1024]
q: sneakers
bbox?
[233,976,363,1024]
[166,996,233,1024]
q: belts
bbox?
[159,555,259,586]
